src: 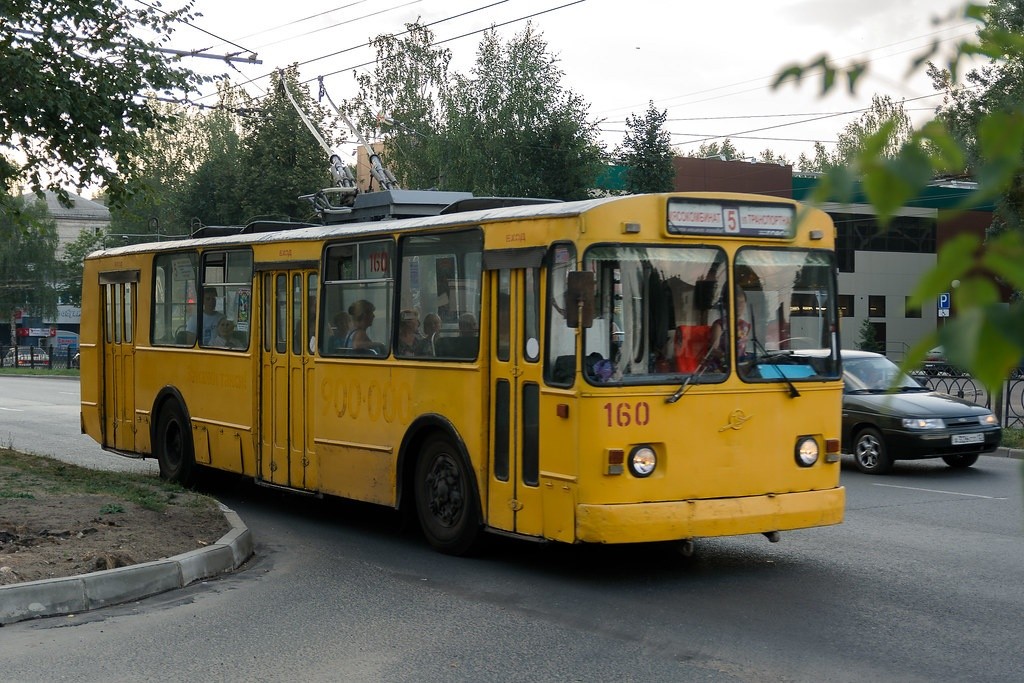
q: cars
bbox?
[2,346,49,369]
[922,345,968,377]
[742,348,1002,475]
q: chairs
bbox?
[331,328,481,360]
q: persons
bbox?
[186,287,225,345]
[444,313,478,359]
[295,295,387,355]
[859,361,887,388]
[208,318,246,348]
[398,310,423,357]
[419,313,443,357]
[710,284,752,374]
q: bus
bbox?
[70,72,848,560]
